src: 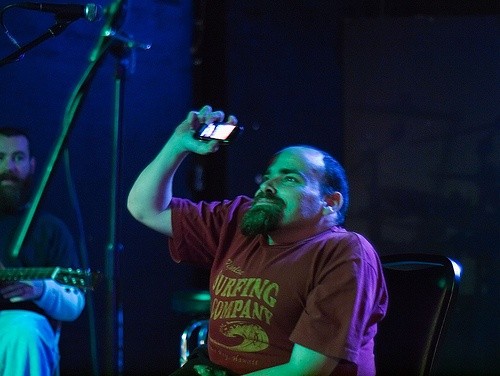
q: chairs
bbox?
[177,252,465,376]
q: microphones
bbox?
[12,3,105,23]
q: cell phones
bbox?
[193,122,244,145]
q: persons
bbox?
[127,105,388,376]
[0,128,87,376]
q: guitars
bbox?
[0,265,102,292]
